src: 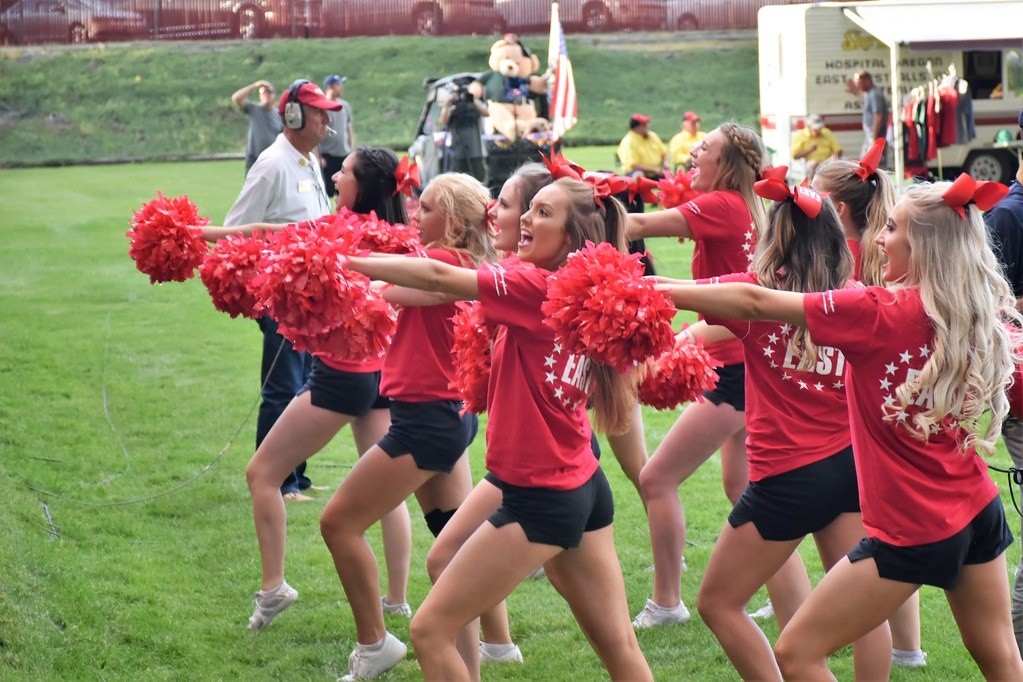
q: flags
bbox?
[546,16,579,139]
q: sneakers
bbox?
[339,633,407,682]
[381,596,411,617]
[748,598,775,620]
[644,557,686,573]
[632,593,690,629]
[248,582,298,631]
[891,652,927,667]
[479,641,524,664]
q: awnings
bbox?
[843,4,1023,186]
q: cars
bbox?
[158,0,799,38]
[0,1,147,44]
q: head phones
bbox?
[285,78,310,129]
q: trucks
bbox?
[757,0,1022,184]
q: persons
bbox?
[440,80,490,182]
[853,71,888,165]
[789,113,842,181]
[190,122,1023,682]
[232,80,284,180]
[617,113,667,181]
[312,74,354,198]
[669,112,709,172]
[223,79,343,500]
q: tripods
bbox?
[441,105,477,178]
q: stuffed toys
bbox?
[479,35,550,141]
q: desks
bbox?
[992,140,1023,167]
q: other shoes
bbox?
[307,485,330,489]
[282,492,313,500]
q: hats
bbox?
[683,112,699,123]
[630,114,652,128]
[278,83,343,115]
[807,114,824,129]
[323,75,347,88]
